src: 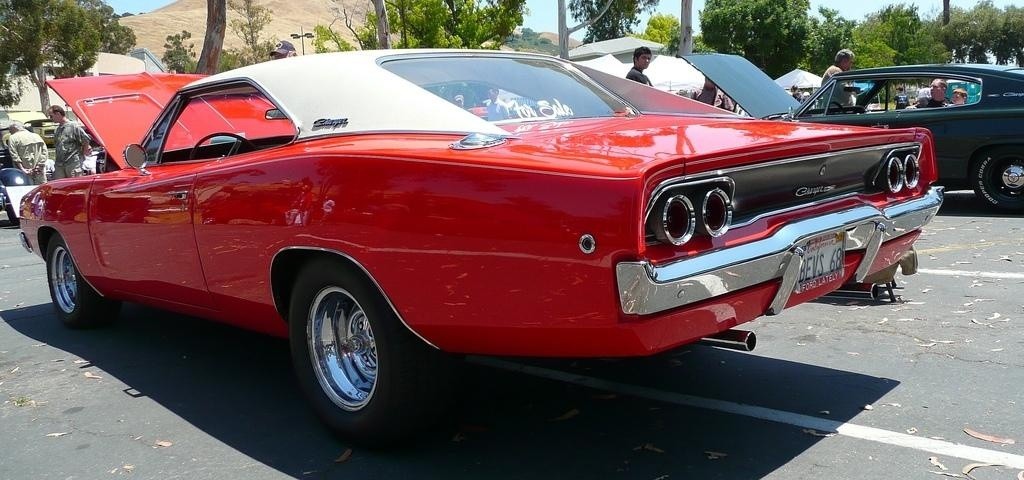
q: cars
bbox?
[682,54,1023,216]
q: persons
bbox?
[905,78,949,109]
[47,104,94,179]
[676,75,750,117]
[791,85,811,104]
[625,46,653,87]
[821,48,857,106]
[8,119,48,186]
[893,86,910,109]
[269,40,297,59]
[951,87,969,104]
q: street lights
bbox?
[290,27,314,55]
